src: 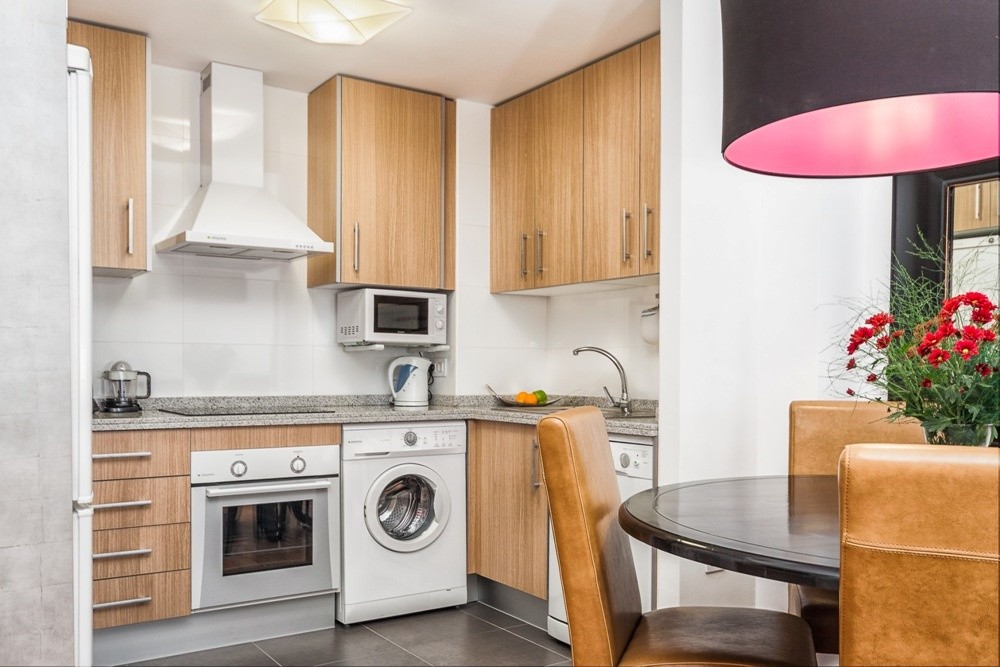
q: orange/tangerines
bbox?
[516,391,537,405]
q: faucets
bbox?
[572,346,634,415]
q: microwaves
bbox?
[335,287,449,347]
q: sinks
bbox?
[488,405,656,419]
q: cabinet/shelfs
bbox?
[464,422,548,604]
[490,66,586,296]
[67,17,152,281]
[306,74,452,293]
[581,32,659,285]
[91,425,194,630]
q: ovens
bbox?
[191,445,343,614]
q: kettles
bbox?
[92,362,152,415]
[386,355,432,407]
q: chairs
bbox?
[534,406,818,665]
[813,440,1000,666]
[788,399,925,615]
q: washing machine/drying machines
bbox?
[334,421,468,627]
[547,434,656,648]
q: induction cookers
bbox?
[155,405,334,415]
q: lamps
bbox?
[720,1,1000,176]
[255,0,415,48]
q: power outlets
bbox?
[427,358,446,377]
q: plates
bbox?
[487,383,560,406]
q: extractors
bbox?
[154,60,334,261]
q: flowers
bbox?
[824,219,1000,447]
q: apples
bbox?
[533,390,546,404]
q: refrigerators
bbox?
[68,42,96,667]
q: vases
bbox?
[920,423,993,446]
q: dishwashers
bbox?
[337,416,469,628]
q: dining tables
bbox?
[620,471,845,653]
